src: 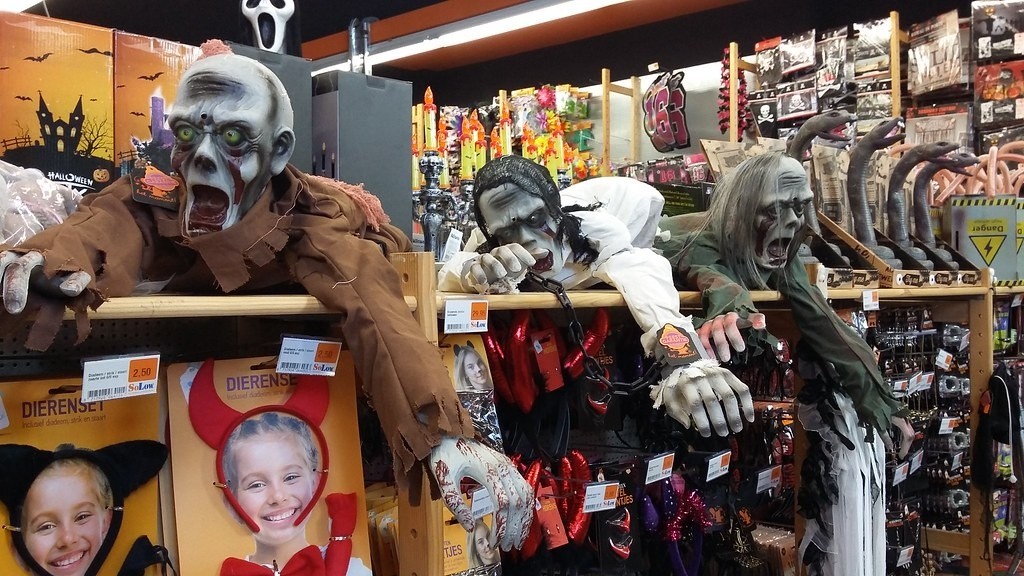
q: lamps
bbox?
[312,0,628,77]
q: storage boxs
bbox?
[313,69,416,243]
[229,41,313,176]
[0,10,114,195]
[113,29,202,181]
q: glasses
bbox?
[727,336,796,510]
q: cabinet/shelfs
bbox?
[0,11,1024,576]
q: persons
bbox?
[4,443,111,576]
[221,411,373,576]
